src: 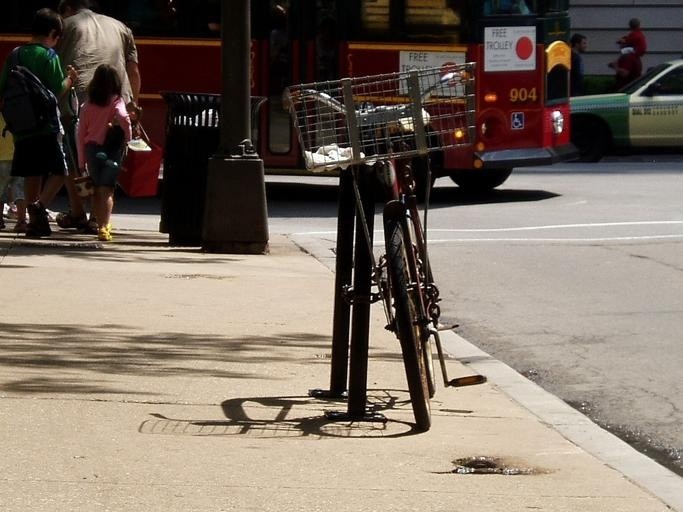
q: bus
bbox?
[0,0,571,199]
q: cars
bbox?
[547,56,683,161]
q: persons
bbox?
[52,0,142,234]
[571,32,588,96]
[608,36,643,92]
[74,64,134,241]
[620,16,647,57]
[8,7,78,239]
[0,111,28,233]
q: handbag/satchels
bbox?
[116,113,165,199]
[103,122,126,165]
[73,163,95,200]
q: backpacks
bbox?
[0,45,61,140]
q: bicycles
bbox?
[283,61,486,430]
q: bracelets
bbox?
[64,75,74,84]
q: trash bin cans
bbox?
[159,92,268,247]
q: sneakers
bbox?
[0,201,52,239]
[76,218,99,235]
[55,210,87,229]
[98,225,112,241]
